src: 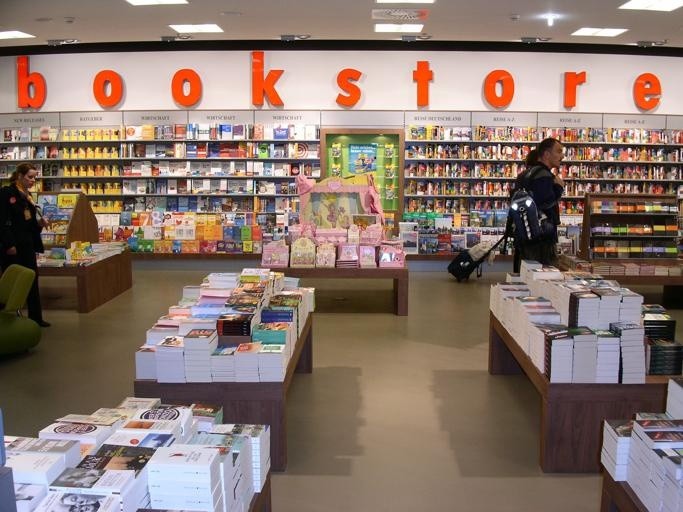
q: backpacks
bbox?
[509,165,555,241]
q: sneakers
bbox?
[38,319,50,327]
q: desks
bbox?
[602,275,683,309]
[39,248,133,312]
[489,308,683,474]
[135,309,314,470]
[260,261,409,317]
[600,465,649,511]
[245,469,273,512]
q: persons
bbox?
[0,162,52,327]
[508,138,565,272]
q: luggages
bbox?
[447,233,510,282]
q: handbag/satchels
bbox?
[469,242,495,265]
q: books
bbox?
[5,397,271,512]
[560,254,682,277]
[489,259,682,383]
[402,126,683,213]
[397,212,584,256]
[600,377,683,511]
[0,124,320,263]
[590,199,679,257]
[135,267,318,384]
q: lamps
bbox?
[533,6,565,27]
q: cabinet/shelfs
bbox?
[406,107,682,260]
[2,106,126,259]
[122,109,323,257]
